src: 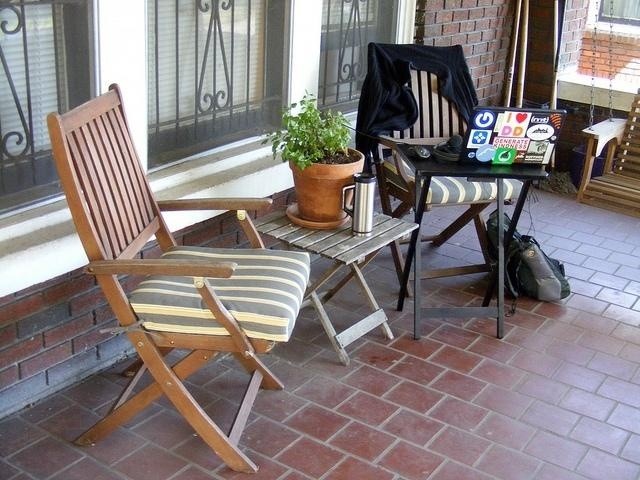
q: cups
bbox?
[341,172,377,237]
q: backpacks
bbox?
[485,207,573,304]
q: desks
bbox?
[391,142,552,340]
[243,211,422,365]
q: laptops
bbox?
[442,106,567,166]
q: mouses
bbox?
[415,146,430,158]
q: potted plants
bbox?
[255,85,367,229]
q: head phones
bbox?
[433,134,462,162]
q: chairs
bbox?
[42,81,313,475]
[360,38,516,297]
[581,85,640,218]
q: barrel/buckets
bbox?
[573,144,605,189]
